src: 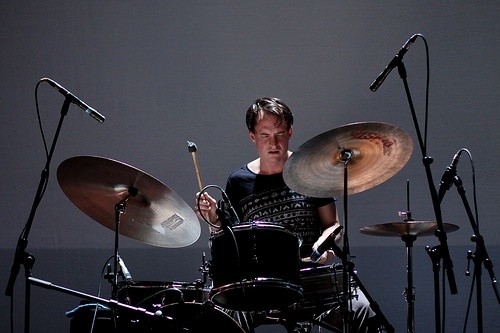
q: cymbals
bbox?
[357,220,461,237]
[281,120,413,198]
[57,155,202,249]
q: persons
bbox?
[194,97,379,333]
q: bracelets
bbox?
[212,217,219,224]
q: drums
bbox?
[206,220,305,311]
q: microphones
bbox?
[118,256,132,281]
[47,79,105,123]
[311,226,344,262]
[369,35,418,92]
[221,190,240,225]
[438,149,464,206]
